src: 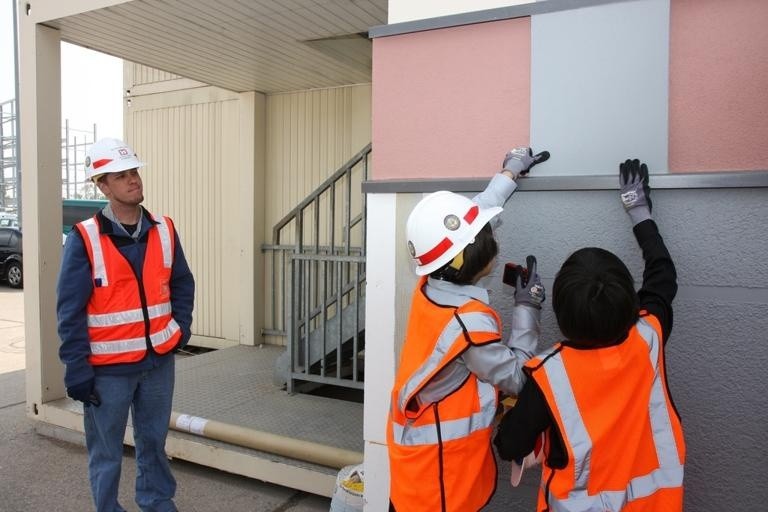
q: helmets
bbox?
[490,394,545,487]
[83,136,146,179]
[405,189,504,278]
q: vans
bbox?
[62,199,107,235]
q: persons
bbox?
[53,137,195,510]
[386,145,551,512]
[493,156,686,512]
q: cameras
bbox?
[503,263,530,289]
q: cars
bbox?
[0,212,23,290]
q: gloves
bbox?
[66,381,101,408]
[493,425,512,462]
[513,254,545,308]
[618,158,653,224]
[499,145,551,179]
